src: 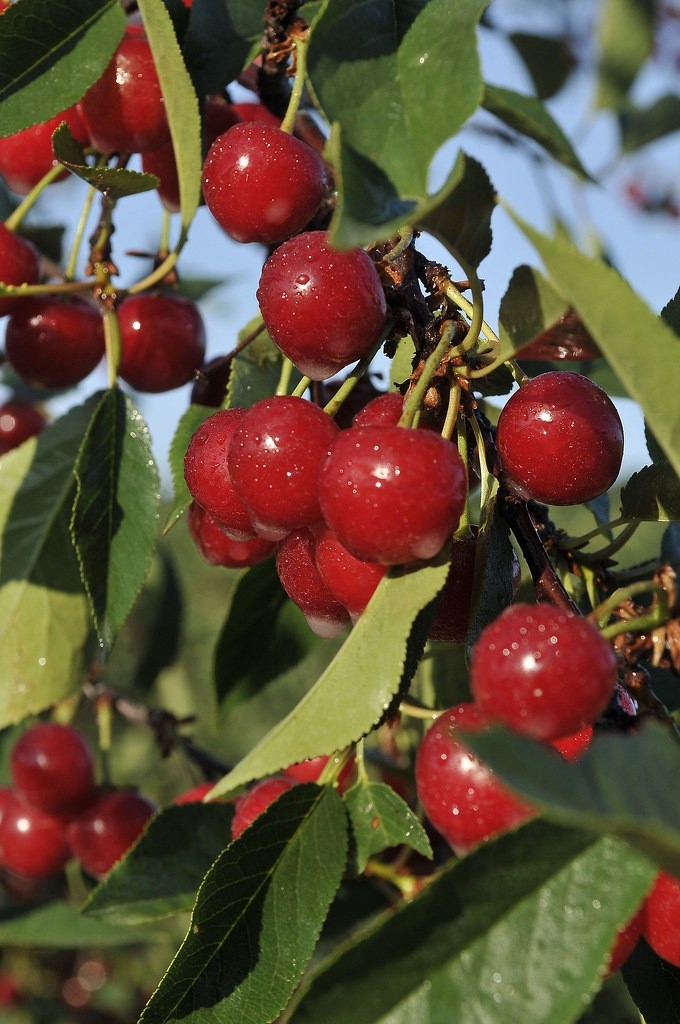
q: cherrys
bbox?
[0,0,680,974]
